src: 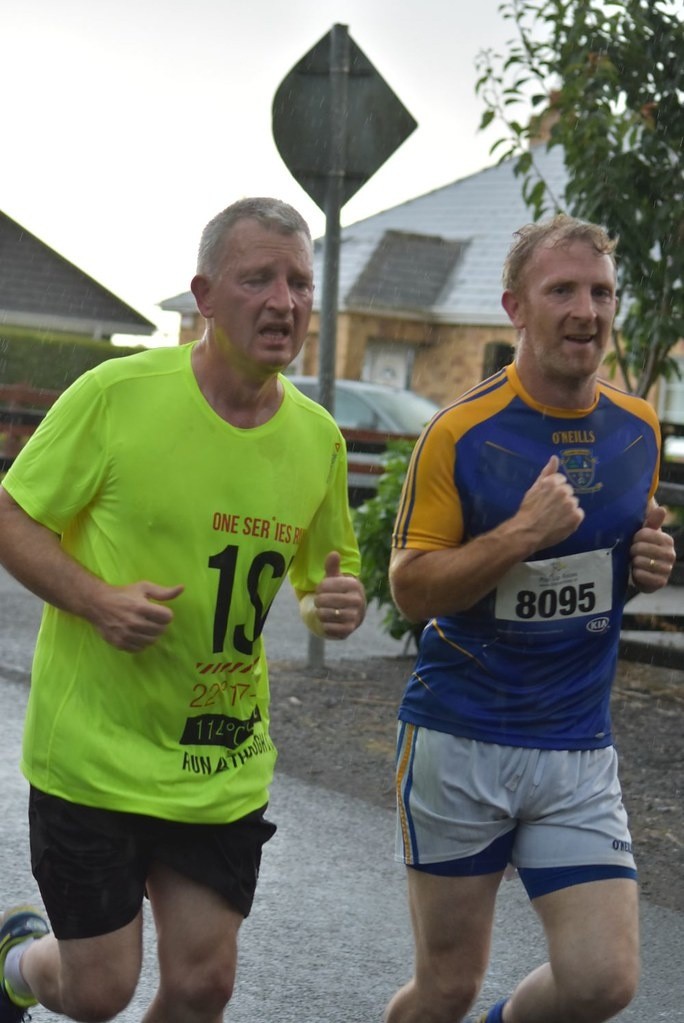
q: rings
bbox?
[648,557,655,573]
[334,608,341,624]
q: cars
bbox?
[285,376,442,498]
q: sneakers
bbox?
[0,903,51,1023]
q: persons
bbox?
[0,198,364,1023]
[367,212,675,1023]
